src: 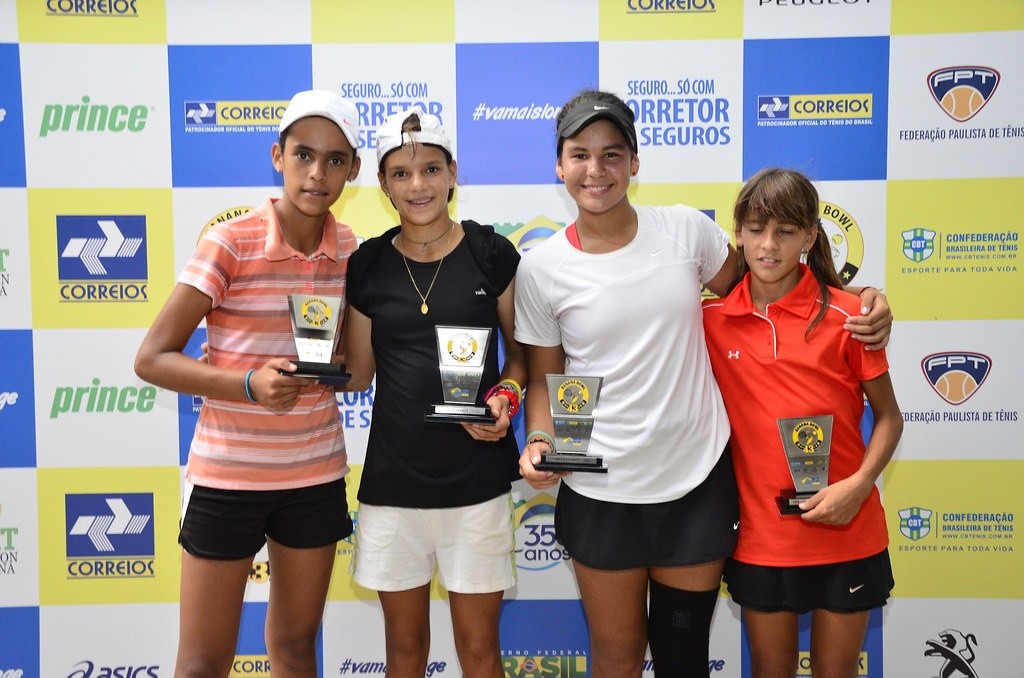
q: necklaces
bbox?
[399,219,455,314]
[577,209,637,247]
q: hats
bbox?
[555,103,637,158]
[277,90,362,149]
[376,107,458,210]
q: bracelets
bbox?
[859,286,876,296]
[523,431,555,453]
[484,380,523,417]
[245,370,260,405]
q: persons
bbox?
[133,91,357,678]
[198,105,526,677]
[515,91,895,678]
[703,167,905,677]
